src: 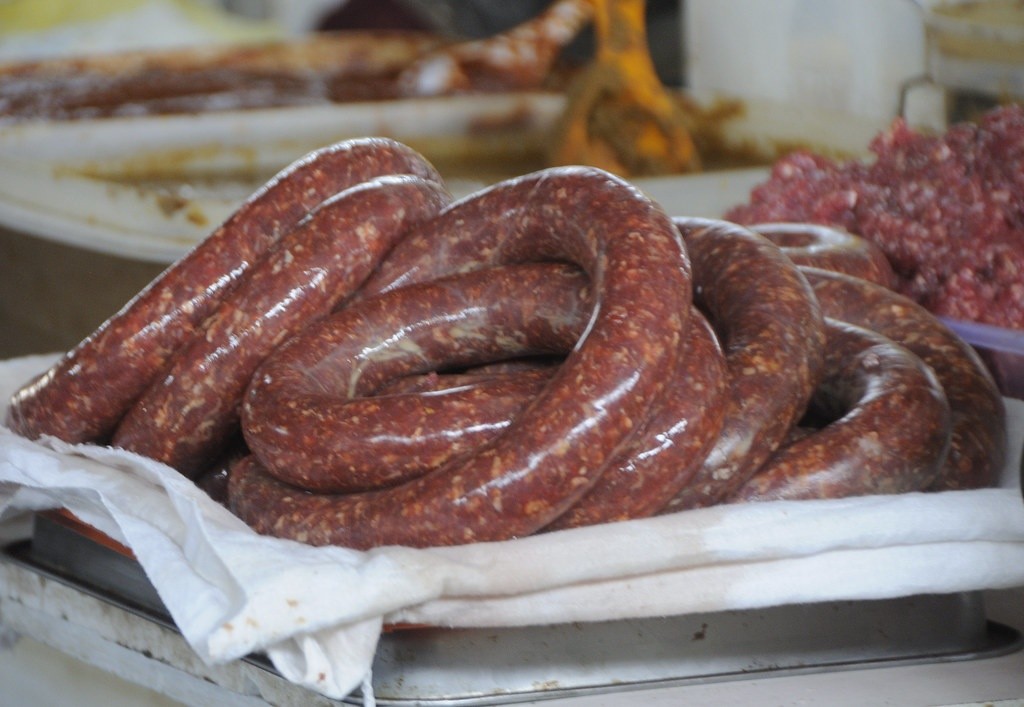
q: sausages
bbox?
[10,136,1008,551]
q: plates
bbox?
[1,3,932,263]
